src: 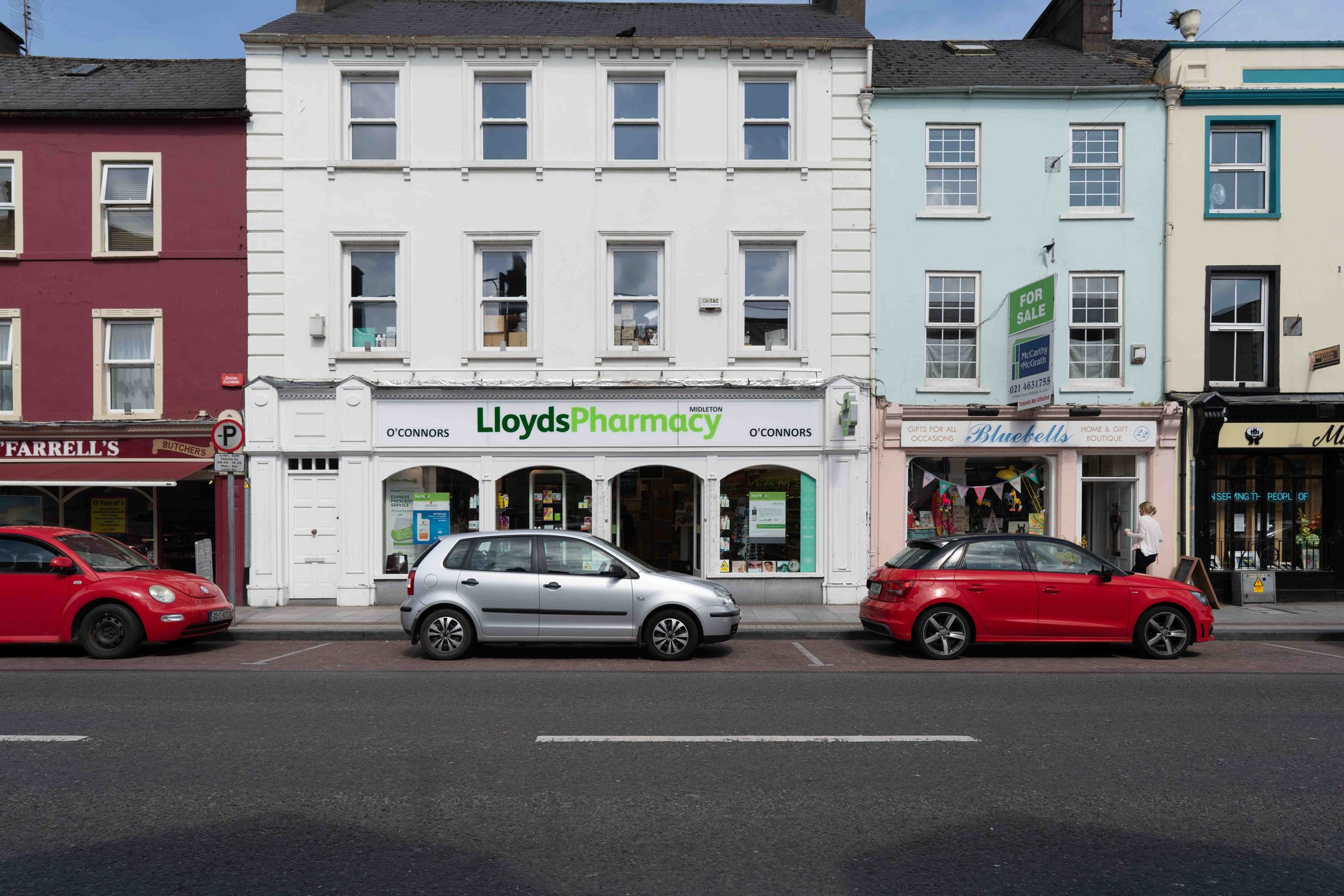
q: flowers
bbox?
[1296,507,1322,547]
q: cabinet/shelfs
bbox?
[468,508,479,530]
[720,507,732,552]
[729,488,801,561]
[578,508,592,531]
[530,470,567,564]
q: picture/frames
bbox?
[1209,555,1224,570]
[1303,549,1319,570]
[1228,551,1260,570]
[920,511,934,527]
[1273,562,1302,570]
[1267,565,1281,570]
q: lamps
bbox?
[967,405,999,416]
[1040,509,1047,513]
[1040,486,1049,491]
[1067,402,1102,417]
[996,465,1042,514]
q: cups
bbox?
[1124,529,1131,537]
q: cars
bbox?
[859,533,1216,661]
[399,529,742,661]
[0,525,235,660]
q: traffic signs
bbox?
[211,419,245,453]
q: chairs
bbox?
[353,328,376,347]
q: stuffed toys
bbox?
[1008,489,1022,512]
[1004,492,1012,500]
[976,498,992,506]
[938,493,951,512]
[1033,487,1041,499]
[946,493,958,503]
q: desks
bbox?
[907,527,936,540]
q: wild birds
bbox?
[616,27,637,37]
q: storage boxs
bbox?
[744,329,788,345]
[578,499,589,508]
[386,326,396,347]
[615,304,647,345]
[484,315,527,347]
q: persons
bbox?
[1123,501,1164,574]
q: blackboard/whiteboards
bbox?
[1172,555,1221,610]
